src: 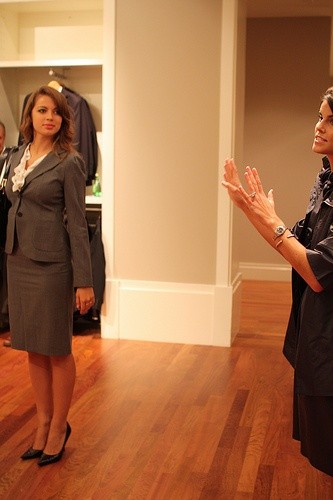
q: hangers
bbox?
[47,70,62,92]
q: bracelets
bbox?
[276,235,298,248]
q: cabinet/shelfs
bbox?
[0,0,105,207]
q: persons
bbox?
[5,91,94,465]
[0,122,16,345]
[223,88,333,474]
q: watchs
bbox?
[271,226,287,242]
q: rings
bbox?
[89,302,92,303]
[86,303,89,305]
[250,191,255,198]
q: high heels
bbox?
[21,445,43,459]
[37,419,72,465]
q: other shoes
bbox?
[4,336,10,346]
